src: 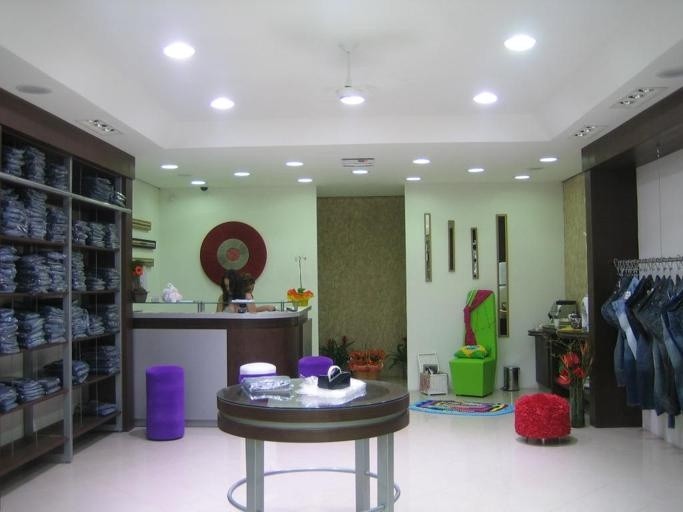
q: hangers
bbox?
[610,254,682,283]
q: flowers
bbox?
[128,260,146,289]
[347,345,386,369]
[549,335,595,388]
[318,335,355,371]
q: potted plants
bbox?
[385,336,406,381]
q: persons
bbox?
[213,269,245,312]
[238,270,277,314]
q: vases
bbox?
[350,366,380,381]
[132,290,147,302]
[569,386,585,429]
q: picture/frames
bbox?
[446,219,456,274]
[493,212,510,338]
[470,226,478,280]
[422,211,433,284]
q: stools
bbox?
[236,362,278,384]
[144,364,186,442]
[295,355,333,377]
[512,393,572,449]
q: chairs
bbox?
[446,288,498,400]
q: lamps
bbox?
[334,40,367,106]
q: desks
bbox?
[215,377,411,512]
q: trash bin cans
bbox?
[503,366,520,391]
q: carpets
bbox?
[409,398,514,418]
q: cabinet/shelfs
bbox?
[0,87,136,483]
[527,325,571,397]
